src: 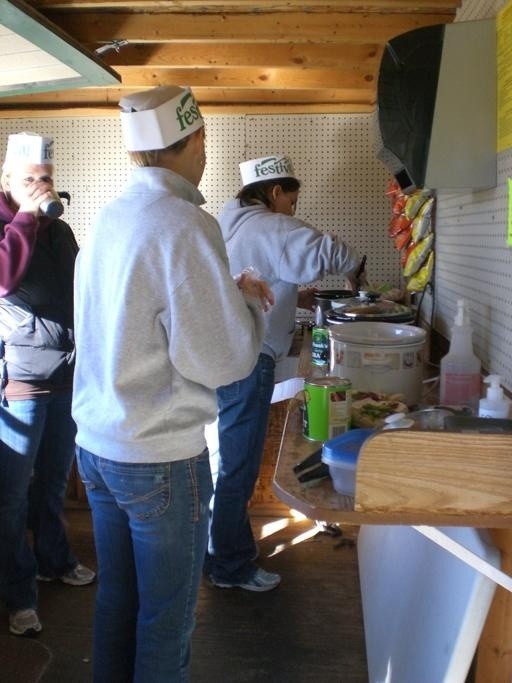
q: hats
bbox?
[117,84,205,151]
[4,131,55,165]
[239,154,295,187]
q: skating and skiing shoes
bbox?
[8,604,42,636]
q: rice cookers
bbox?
[328,322,428,409]
[325,293,416,327]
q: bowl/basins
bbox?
[321,428,384,499]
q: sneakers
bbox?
[34,558,95,586]
[207,545,282,592]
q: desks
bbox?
[271,315,512,590]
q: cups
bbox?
[38,188,72,220]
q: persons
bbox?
[210,159,366,591]
[1,130,96,634]
[72,88,273,682]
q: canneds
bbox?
[301,376,352,442]
[312,325,330,366]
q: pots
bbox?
[314,288,360,329]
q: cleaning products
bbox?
[478,374,511,420]
